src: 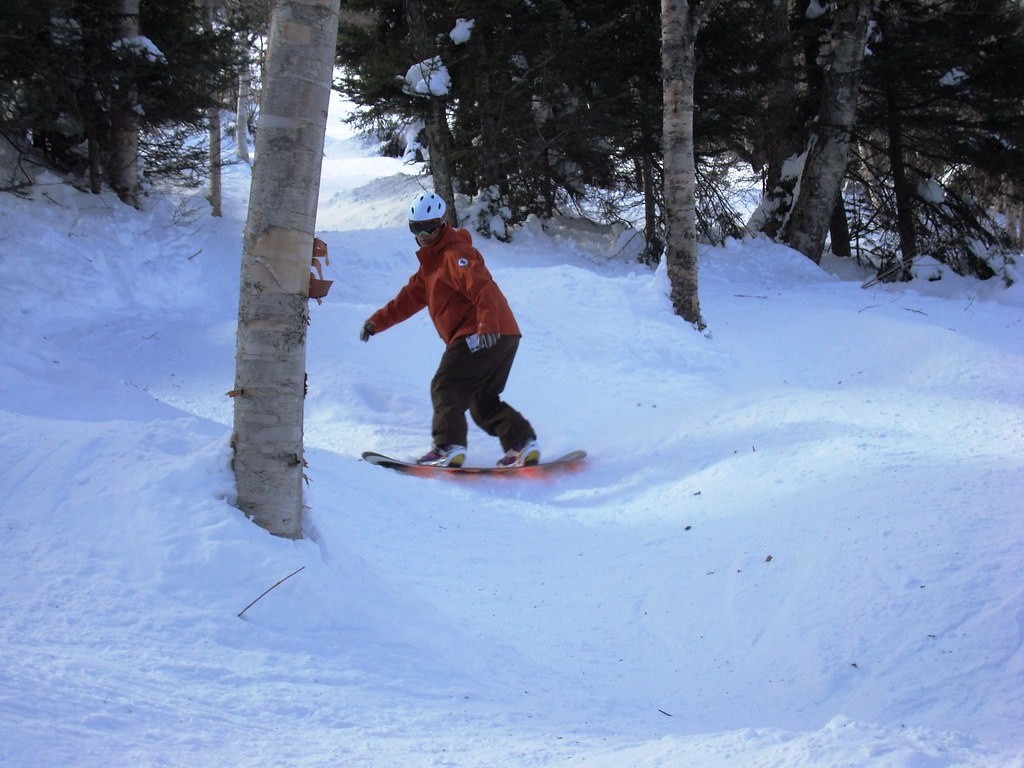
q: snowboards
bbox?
[362,450,588,481]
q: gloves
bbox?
[478,332,500,348]
[359,321,373,342]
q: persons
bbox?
[359,192,542,472]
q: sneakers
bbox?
[417,444,466,467]
[497,438,540,467]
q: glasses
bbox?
[410,221,444,235]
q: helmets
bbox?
[409,192,446,221]
